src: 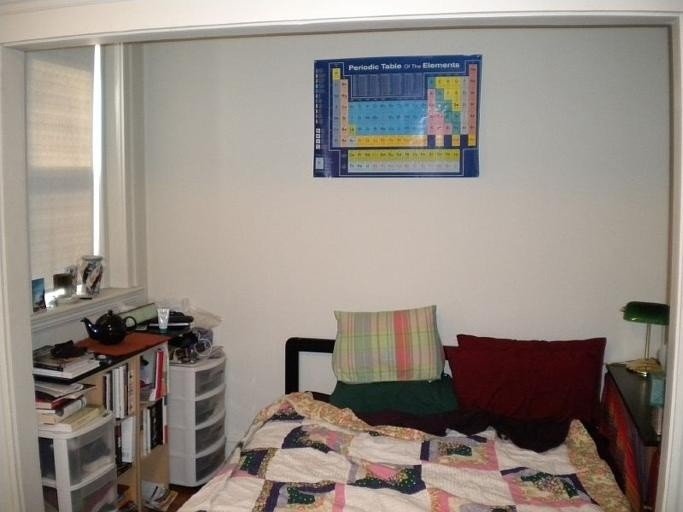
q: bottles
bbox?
[80,255,103,296]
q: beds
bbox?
[173,338,634,510]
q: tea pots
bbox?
[79,309,137,344]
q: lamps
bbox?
[619,300,670,377]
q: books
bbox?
[35,349,177,512]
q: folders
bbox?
[142,351,164,401]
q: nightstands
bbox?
[601,362,667,511]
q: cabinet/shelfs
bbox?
[169,349,227,486]
[32,331,171,512]
[38,410,118,511]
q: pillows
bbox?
[327,371,459,415]
[457,333,610,426]
[332,304,443,384]
[441,343,460,392]
